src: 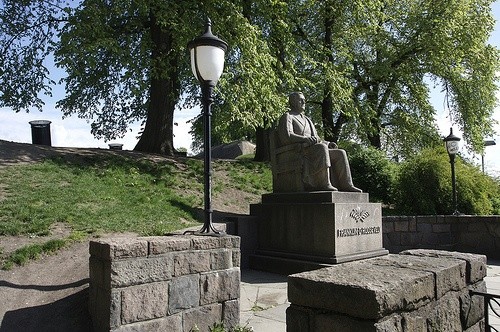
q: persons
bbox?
[278,92,363,192]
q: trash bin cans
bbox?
[109,143,124,150]
[30,119,52,145]
